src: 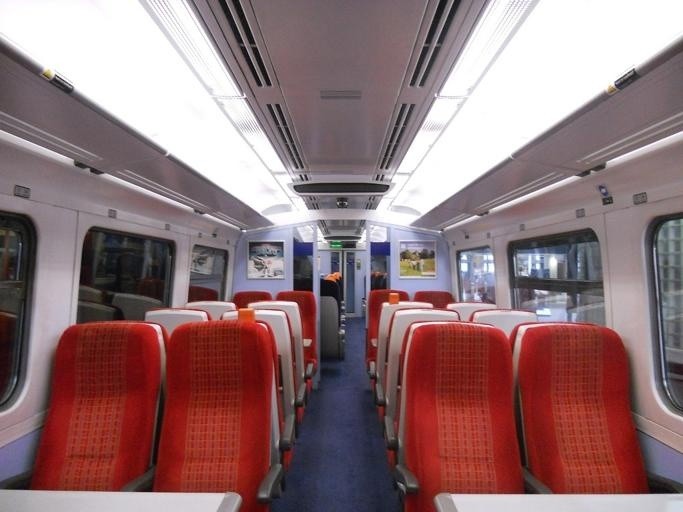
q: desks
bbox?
[1,487,244,508]
[427,492,680,508]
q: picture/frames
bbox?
[396,240,437,279]
[245,239,285,280]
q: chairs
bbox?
[391,316,529,507]
[150,317,285,508]
[359,284,607,472]
[19,318,168,490]
[141,274,349,472]
[515,321,653,498]
